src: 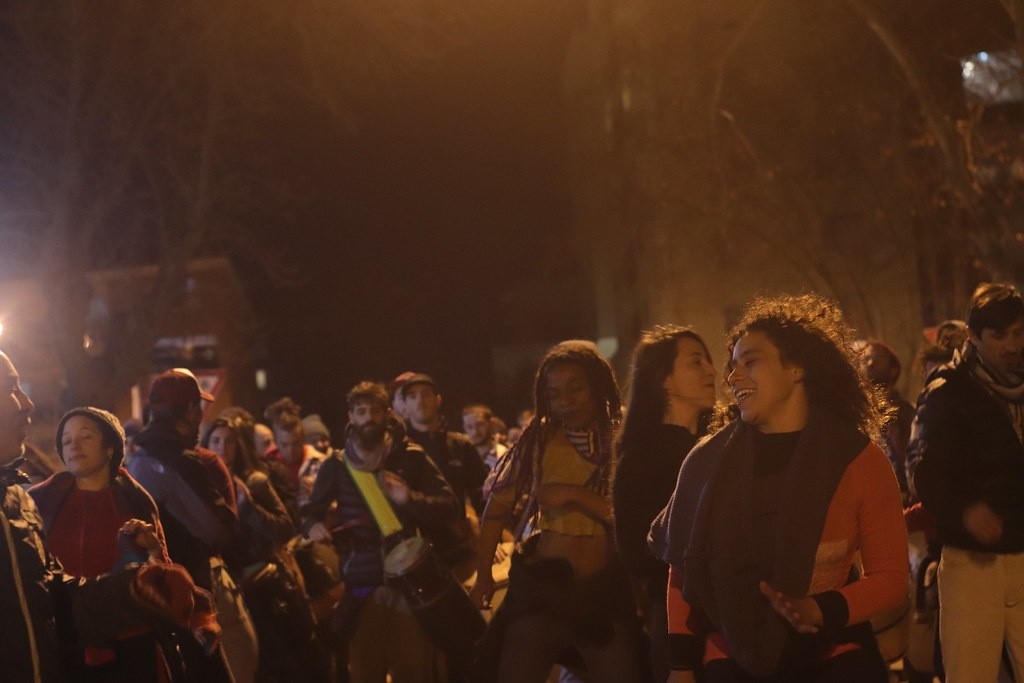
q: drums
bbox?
[382,535,491,658]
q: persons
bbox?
[859,320,967,683]
[468,340,622,683]
[125,368,242,682]
[647,293,909,683]
[200,398,340,683]
[391,371,534,584]
[0,350,196,683]
[904,282,1024,683]
[612,327,718,683]
[26,406,173,683]
[303,382,460,683]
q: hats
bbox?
[150,366,216,403]
[401,374,438,395]
[346,379,391,402]
[56,406,126,469]
